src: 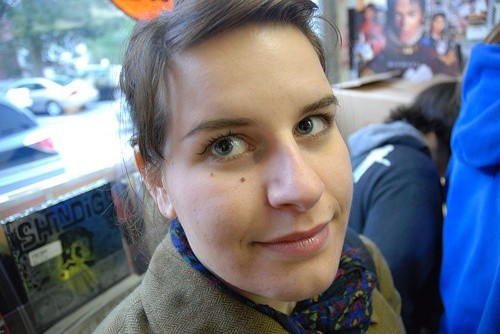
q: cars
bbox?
[0,63,126,115]
[0,97,79,204]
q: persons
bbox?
[437,20,499,334]
[351,0,462,81]
[348,80,463,334]
[91,0,407,334]
